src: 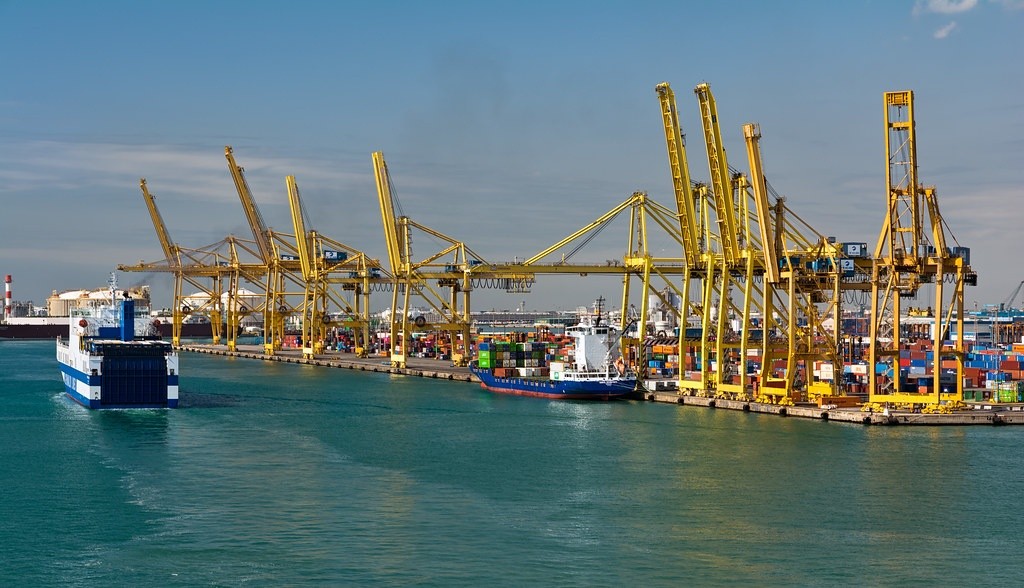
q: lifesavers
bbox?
[186,348,189,351]
[223,352,226,355]
[419,372,423,377]
[174,347,177,350]
[466,378,471,382]
[433,374,436,378]
[306,361,309,364]
[203,350,206,353]
[349,366,353,369]
[237,354,240,357]
[253,356,256,359]
[779,409,787,416]
[287,359,290,362]
[180,348,183,351]
[678,399,684,405]
[863,417,871,425]
[327,363,330,367]
[316,362,319,366]
[216,351,219,354]
[261,356,264,360]
[192,349,195,352]
[269,357,272,361]
[449,375,453,380]
[709,402,715,407]
[338,365,341,368]
[278,359,281,362]
[648,395,654,402]
[374,369,377,372]
[210,351,212,354]
[197,350,200,352]
[821,413,828,419]
[245,355,248,358]
[387,370,390,373]
[231,353,233,356]
[743,405,750,412]
[361,367,365,371]
[296,360,299,363]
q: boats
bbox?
[0,274,244,338]
[467,294,637,403]
[55,270,180,411]
